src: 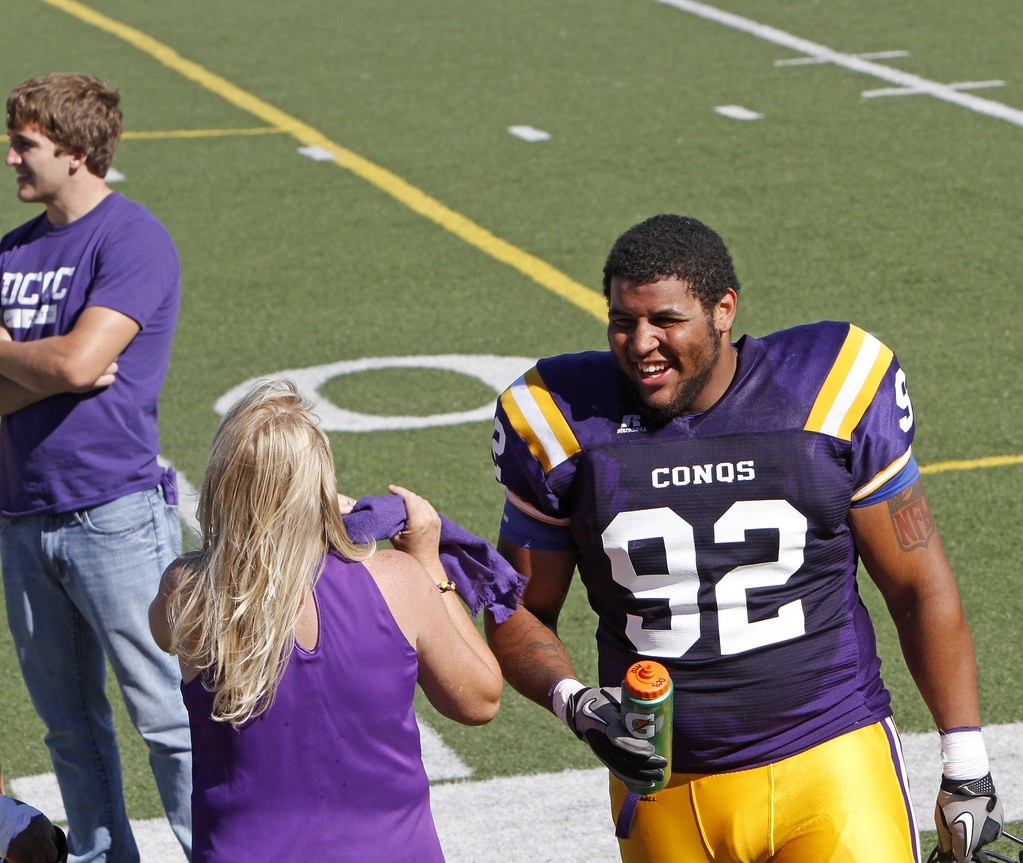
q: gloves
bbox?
[550,677,666,789]
[935,726,1005,863]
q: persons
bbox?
[483,214,1006,863]
[149,376,504,863]
[0,73,194,863]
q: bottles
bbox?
[621,661,672,794]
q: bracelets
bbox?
[438,580,456,593]
